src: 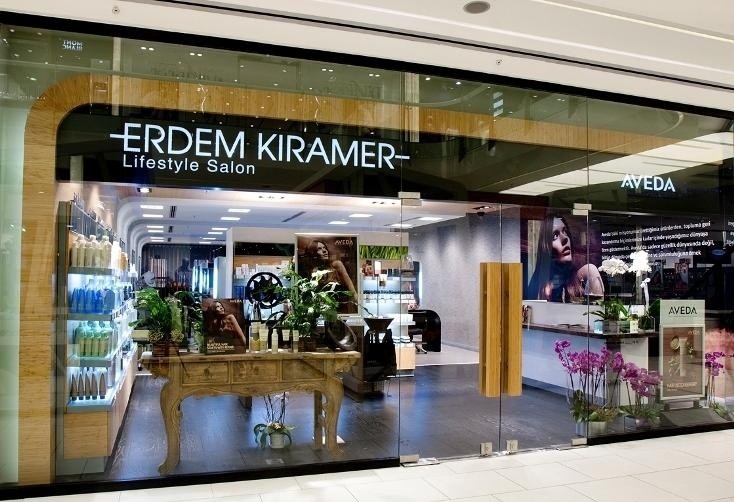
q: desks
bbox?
[142,350,362,478]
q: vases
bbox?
[576,414,660,435]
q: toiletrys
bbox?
[69,372,107,399]
[68,234,138,401]
[258,329,269,352]
[292,329,300,353]
[70,233,138,369]
[282,329,291,349]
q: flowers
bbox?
[704,350,729,410]
[551,338,664,425]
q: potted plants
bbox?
[584,296,629,332]
[255,268,355,352]
[251,392,294,449]
[129,288,197,357]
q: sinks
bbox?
[365,318,394,331]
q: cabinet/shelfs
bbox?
[55,201,139,477]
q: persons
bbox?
[305,240,358,314]
[528,216,605,303]
[201,302,246,349]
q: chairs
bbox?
[408,312,430,354]
